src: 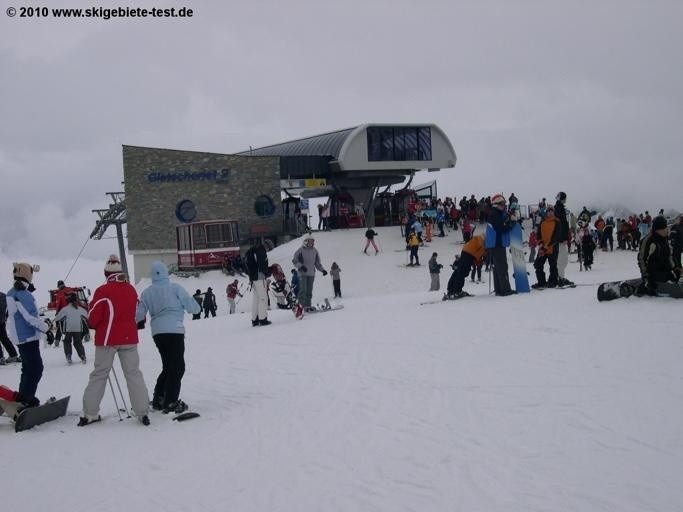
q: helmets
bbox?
[13,262,33,284]
[490,193,505,207]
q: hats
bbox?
[546,206,555,212]
[652,216,668,231]
[265,239,273,250]
[103,254,121,277]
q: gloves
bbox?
[46,330,53,344]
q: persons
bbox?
[77,254,151,425]
[4,262,51,400]
[134,262,202,413]
[0,192,683,365]
[0,384,37,420]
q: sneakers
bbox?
[152,397,187,410]
[532,282,545,289]
[0,356,17,365]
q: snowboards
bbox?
[509,222,529,293]
[15,396,71,433]
[597,278,646,302]
[271,264,304,320]
[148,399,199,420]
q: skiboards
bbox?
[395,231,448,252]
[399,263,425,268]
[362,250,378,256]
[60,408,157,433]
[277,303,344,314]
[576,232,583,270]
[420,294,475,305]
[469,279,486,285]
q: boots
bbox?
[252,315,271,326]
